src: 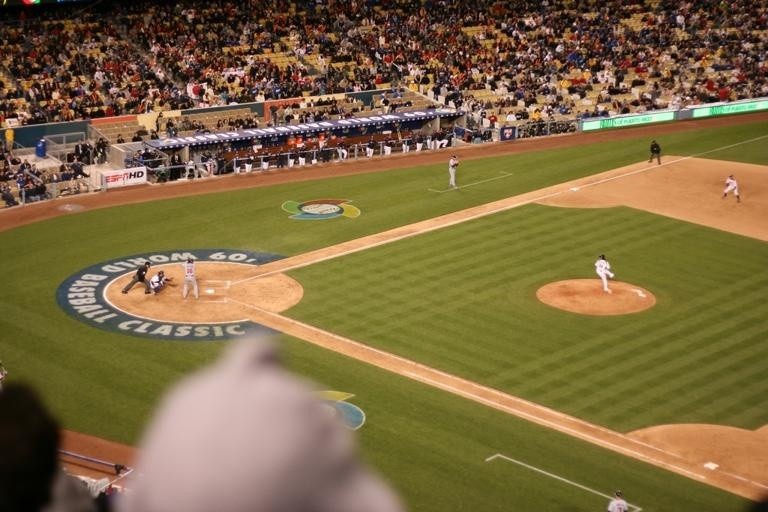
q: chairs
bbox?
[92,108,268,143]
[296,91,436,121]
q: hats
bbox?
[111,335,406,510]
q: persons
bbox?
[0,329,403,512]
[122,262,151,294]
[150,271,178,294]
[595,254,615,293]
[0,0,768,208]
[183,258,199,299]
[607,490,628,512]
